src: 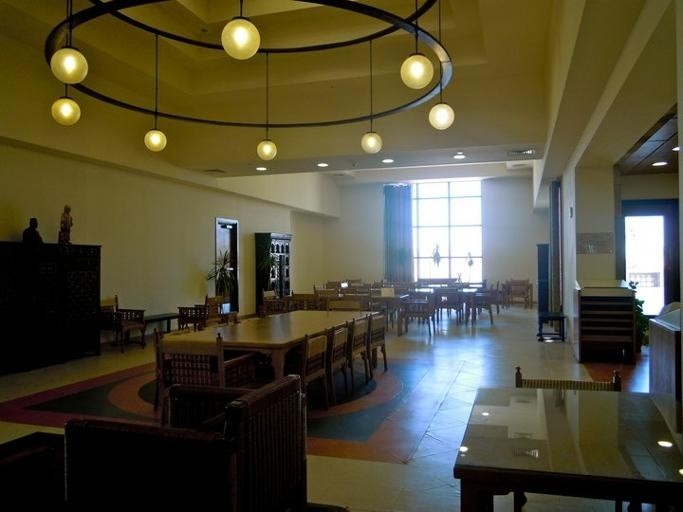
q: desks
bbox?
[456,383,681,511]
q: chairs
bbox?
[95,291,145,355]
[59,370,309,511]
[151,275,393,426]
[394,276,535,338]
[514,364,626,512]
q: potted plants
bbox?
[204,246,234,304]
[258,246,276,299]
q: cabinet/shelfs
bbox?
[573,278,642,370]
[253,229,293,314]
[0,242,104,379]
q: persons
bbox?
[57,205,72,244]
[21,217,44,243]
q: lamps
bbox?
[45,0,456,162]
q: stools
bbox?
[537,310,565,343]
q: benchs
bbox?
[136,310,179,339]
